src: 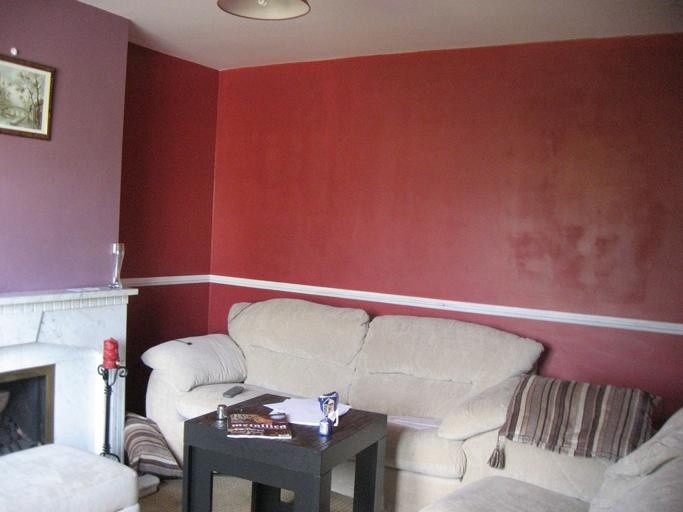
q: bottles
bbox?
[216,404,227,420]
[319,416,334,435]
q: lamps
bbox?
[216,0,311,21]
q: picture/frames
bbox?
[0,53,58,141]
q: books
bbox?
[225,406,293,441]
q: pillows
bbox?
[122,413,182,479]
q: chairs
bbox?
[416,374,661,512]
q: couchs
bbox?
[142,299,547,511]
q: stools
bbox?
[0,441,140,512]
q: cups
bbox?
[108,243,126,288]
